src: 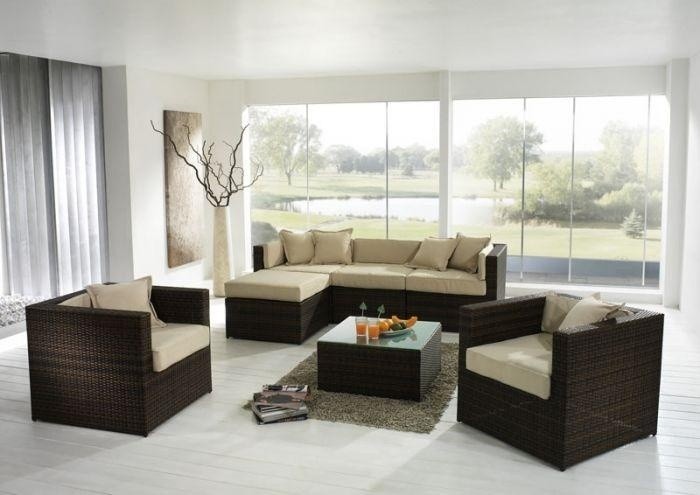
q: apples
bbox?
[379,319,393,331]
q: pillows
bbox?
[85,277,168,331]
[279,229,314,265]
[558,292,624,328]
[310,229,354,264]
[449,232,489,274]
[404,237,458,271]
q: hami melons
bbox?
[392,315,417,328]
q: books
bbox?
[250,383,312,424]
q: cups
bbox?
[354,316,368,337]
[366,318,380,340]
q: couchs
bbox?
[253,237,507,332]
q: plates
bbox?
[379,324,414,337]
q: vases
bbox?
[213,206,234,297]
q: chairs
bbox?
[26,282,212,437]
[457,290,664,471]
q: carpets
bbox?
[242,342,458,435]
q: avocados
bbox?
[400,321,407,329]
[391,323,402,331]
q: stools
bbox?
[224,268,330,344]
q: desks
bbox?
[317,316,442,403]
